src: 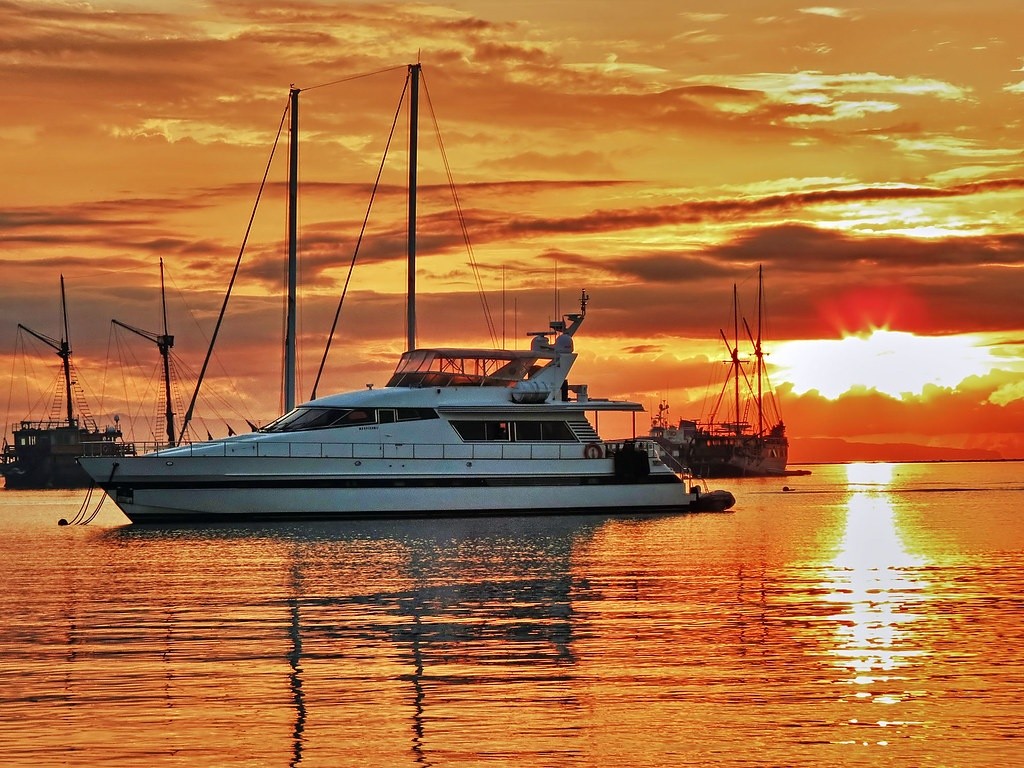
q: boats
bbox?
[75,312,736,516]
[0,271,133,490]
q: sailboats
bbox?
[648,258,790,475]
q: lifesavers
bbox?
[584,442,603,459]
